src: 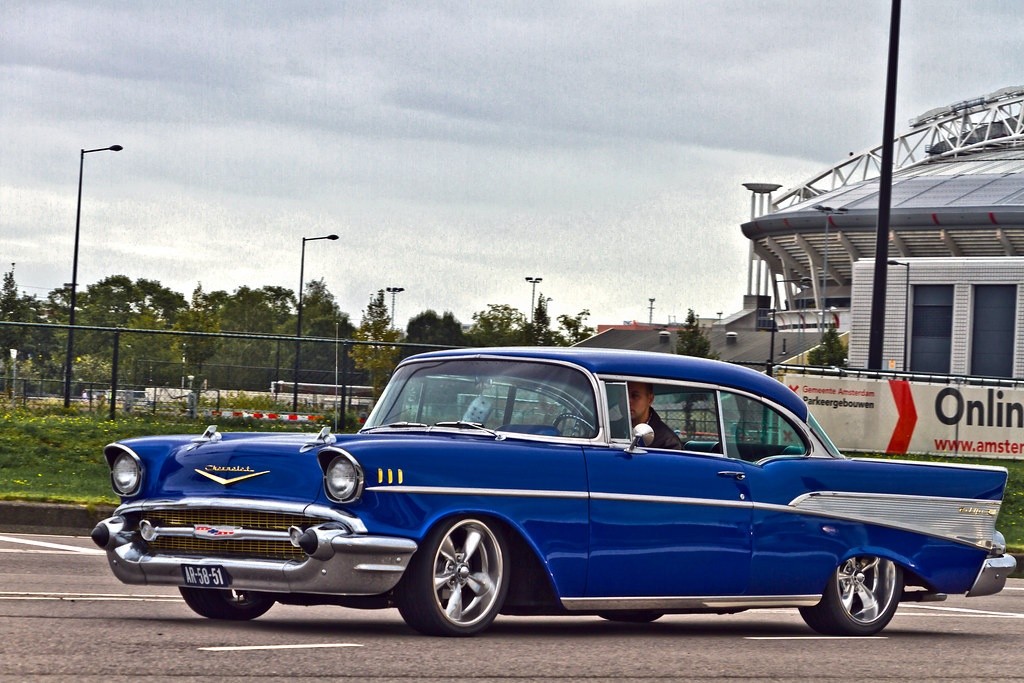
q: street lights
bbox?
[888,259,909,380]
[526,277,543,321]
[64,145,125,407]
[293,234,339,413]
[765,308,776,375]
[387,288,406,332]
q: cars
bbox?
[90,346,1016,637]
[81,389,106,400]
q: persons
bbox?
[610,381,681,451]
